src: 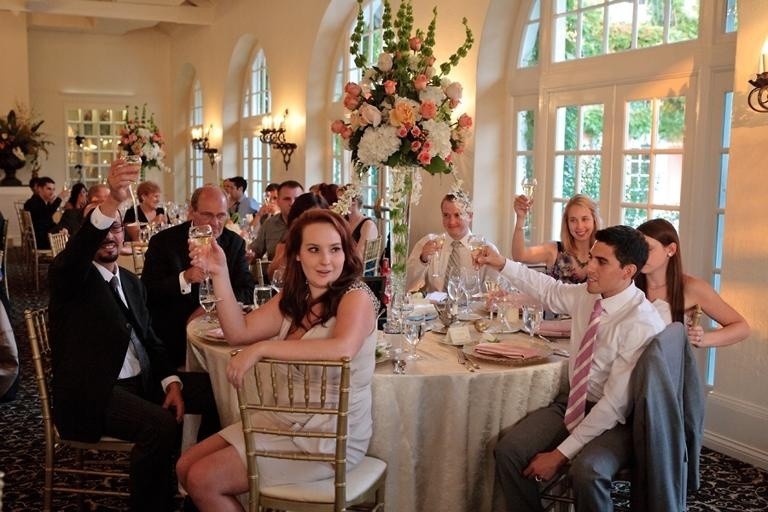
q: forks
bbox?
[455,349,474,371]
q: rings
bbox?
[534,475,543,484]
[697,336,702,342]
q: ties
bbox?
[562,298,602,435]
[442,241,461,293]
[109,275,151,371]
[233,202,239,223]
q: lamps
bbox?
[191,126,217,169]
[747,54,768,113]
[259,115,296,171]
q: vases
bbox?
[139,165,145,182]
[0,146,27,186]
[383,166,415,334]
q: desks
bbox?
[0,187,34,248]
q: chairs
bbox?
[14,201,49,290]
[256,214,383,305]
[24,303,136,512]
[48,230,69,257]
[538,321,704,506]
[230,349,387,512]
[0,214,13,301]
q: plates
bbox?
[194,323,227,343]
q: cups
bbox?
[239,213,264,253]
[138,202,189,246]
[271,270,285,294]
[254,284,272,308]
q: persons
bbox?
[1,172,381,283]
[46,155,222,512]
[634,218,753,350]
[464,224,670,512]
[138,181,259,371]
[173,204,381,511]
[510,192,603,320]
[402,190,501,301]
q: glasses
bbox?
[109,223,124,234]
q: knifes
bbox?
[539,334,568,354]
[463,352,481,369]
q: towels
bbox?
[205,327,224,339]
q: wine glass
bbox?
[431,232,446,277]
[123,154,150,227]
[374,268,552,365]
[263,190,272,221]
[198,284,218,323]
[188,224,223,304]
[466,233,489,297]
[63,178,72,202]
[520,177,539,229]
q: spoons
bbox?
[391,354,405,374]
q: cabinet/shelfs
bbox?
[64,103,126,192]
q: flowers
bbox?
[330,0,474,275]
[118,101,173,181]
[0,95,55,170]
